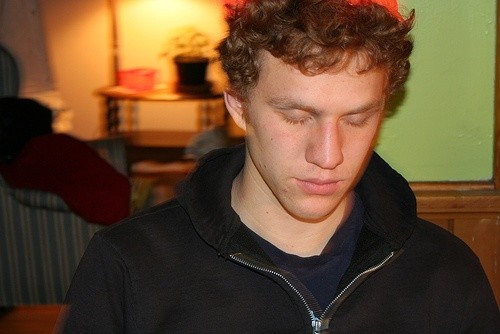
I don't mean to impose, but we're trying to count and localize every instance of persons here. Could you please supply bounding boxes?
[0,97,131,226]
[64,0,500,334]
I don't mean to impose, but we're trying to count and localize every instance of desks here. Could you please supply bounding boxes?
[93,79,231,148]
